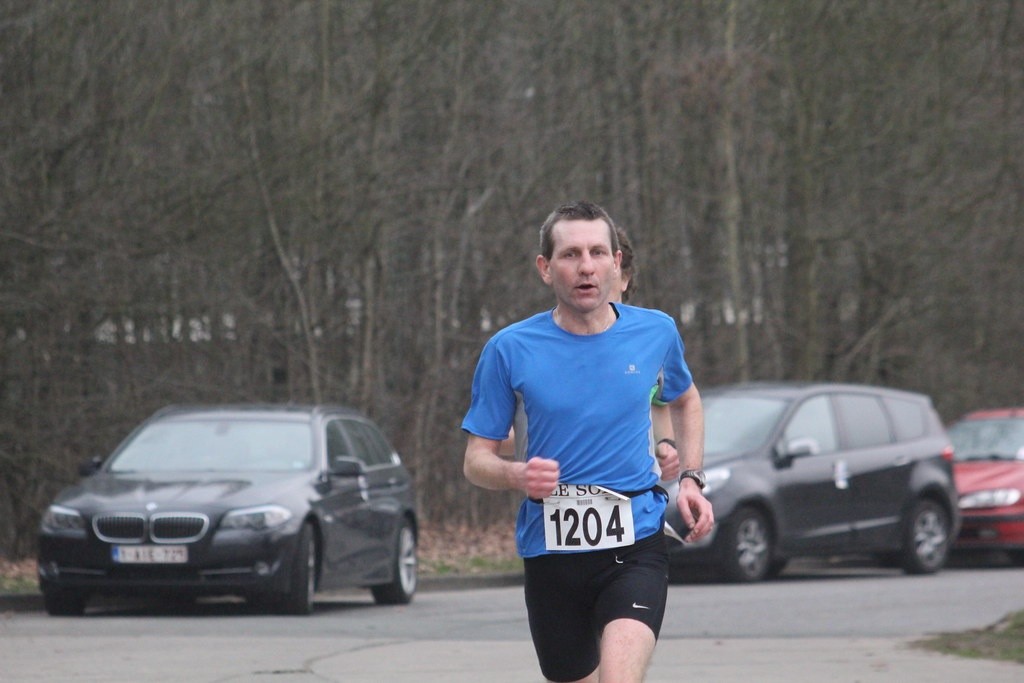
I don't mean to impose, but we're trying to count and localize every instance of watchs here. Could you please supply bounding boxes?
[678,469,707,489]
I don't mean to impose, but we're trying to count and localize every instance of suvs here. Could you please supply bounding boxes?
[645,378,961,586]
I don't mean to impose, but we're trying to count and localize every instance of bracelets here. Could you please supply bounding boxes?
[657,438,676,449]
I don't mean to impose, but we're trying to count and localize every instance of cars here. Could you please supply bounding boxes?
[945,406,1024,572]
[32,397,418,616]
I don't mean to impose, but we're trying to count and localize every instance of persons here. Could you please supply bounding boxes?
[462,201,715,683]
